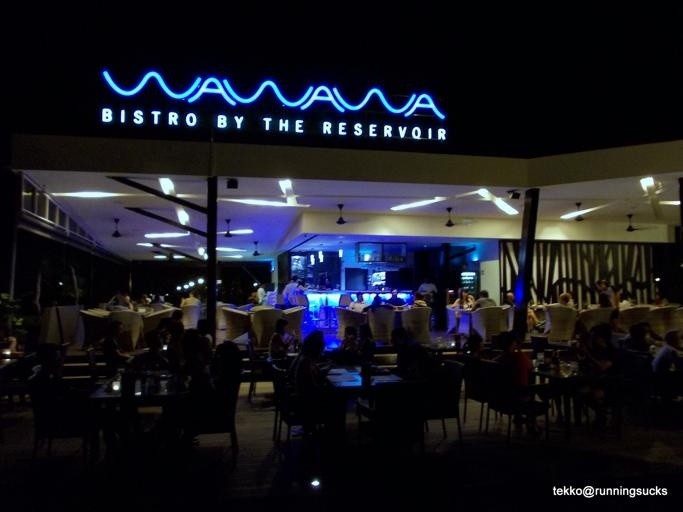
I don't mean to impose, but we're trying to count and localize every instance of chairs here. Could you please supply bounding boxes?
[81,302,178,348]
[262,357,299,439]
[650,303,679,340]
[618,303,650,330]
[165,339,245,450]
[338,294,355,307]
[424,359,465,444]
[181,304,201,332]
[367,309,395,344]
[545,303,578,346]
[471,305,503,340]
[336,306,367,340]
[580,306,616,331]
[283,306,308,342]
[401,307,432,342]
[250,306,282,346]
[219,306,249,340]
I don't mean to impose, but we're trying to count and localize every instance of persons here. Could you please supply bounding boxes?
[388,287,406,305]
[181,291,200,307]
[158,310,184,345]
[452,289,474,308]
[366,297,396,310]
[0,333,17,353]
[104,320,132,377]
[492,331,549,427]
[339,327,357,351]
[145,324,166,350]
[464,335,484,358]
[355,324,376,367]
[104,290,131,305]
[411,293,428,308]
[350,292,367,310]
[418,276,438,314]
[613,285,625,332]
[652,293,669,306]
[583,334,637,407]
[354,329,451,458]
[471,291,496,311]
[283,276,298,308]
[140,294,153,305]
[143,341,242,462]
[174,331,221,385]
[289,331,334,386]
[637,322,663,345]
[27,343,117,465]
[624,326,650,352]
[196,319,212,348]
[291,279,308,306]
[558,293,572,306]
[597,280,615,307]
[588,325,636,364]
[506,292,547,327]
[320,278,332,290]
[151,296,163,310]
[258,281,271,304]
[652,330,683,376]
[270,318,296,360]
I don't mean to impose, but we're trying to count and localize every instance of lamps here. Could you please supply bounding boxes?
[625,214,638,234]
[574,199,584,222]
[318,244,324,263]
[338,241,343,258]
[253,240,262,257]
[310,246,315,266]
[335,203,347,225]
[224,220,234,238]
[110,215,123,239]
[446,206,455,228]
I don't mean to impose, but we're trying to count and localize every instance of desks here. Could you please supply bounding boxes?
[90,371,179,414]
[530,360,581,417]
[344,303,407,315]
[320,367,402,427]
